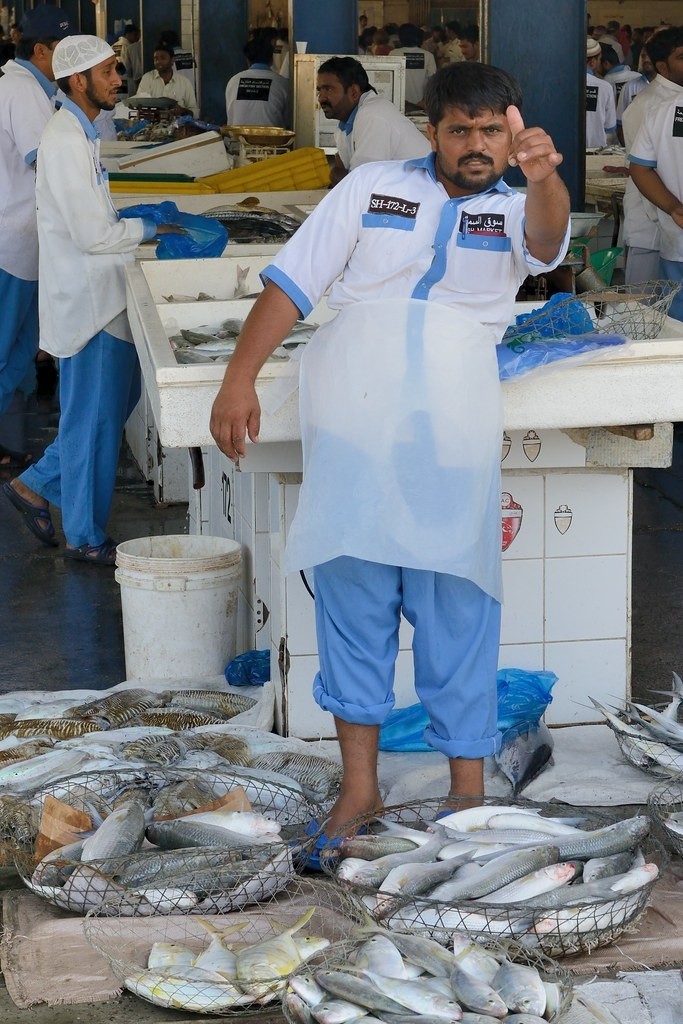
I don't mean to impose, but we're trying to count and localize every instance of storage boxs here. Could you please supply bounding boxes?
[101,130,683,447]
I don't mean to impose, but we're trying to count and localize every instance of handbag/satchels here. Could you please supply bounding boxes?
[119,201,229,260]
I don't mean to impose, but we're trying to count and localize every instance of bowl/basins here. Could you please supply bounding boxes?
[568,236,624,288]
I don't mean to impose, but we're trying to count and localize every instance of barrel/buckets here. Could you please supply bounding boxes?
[114,534,243,683]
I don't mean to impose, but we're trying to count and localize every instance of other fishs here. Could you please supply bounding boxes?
[0,671,683,1024]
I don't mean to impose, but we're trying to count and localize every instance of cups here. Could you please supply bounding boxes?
[296,41,308,55]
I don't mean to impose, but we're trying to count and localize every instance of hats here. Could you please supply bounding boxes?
[21,4,74,36]
[586,38,601,57]
[420,25,432,32]
[52,35,116,80]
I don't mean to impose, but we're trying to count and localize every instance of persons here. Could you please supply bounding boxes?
[317,57,432,186]
[586,21,655,148]
[0,5,184,564]
[209,64,571,869]
[136,45,201,119]
[225,27,290,147]
[358,15,480,113]
[622,29,683,322]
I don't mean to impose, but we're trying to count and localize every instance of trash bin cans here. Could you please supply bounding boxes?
[115,535,243,679]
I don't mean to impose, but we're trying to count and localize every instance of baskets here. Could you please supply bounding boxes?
[606,702,683,771]
[505,279,681,356]
[651,783,683,848]
[81,872,367,1013]
[6,766,327,915]
[280,930,575,1024]
[321,794,670,954]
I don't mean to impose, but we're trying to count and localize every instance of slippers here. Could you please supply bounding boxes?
[299,815,370,871]
[3,481,58,547]
[0,453,33,468]
[62,538,122,565]
